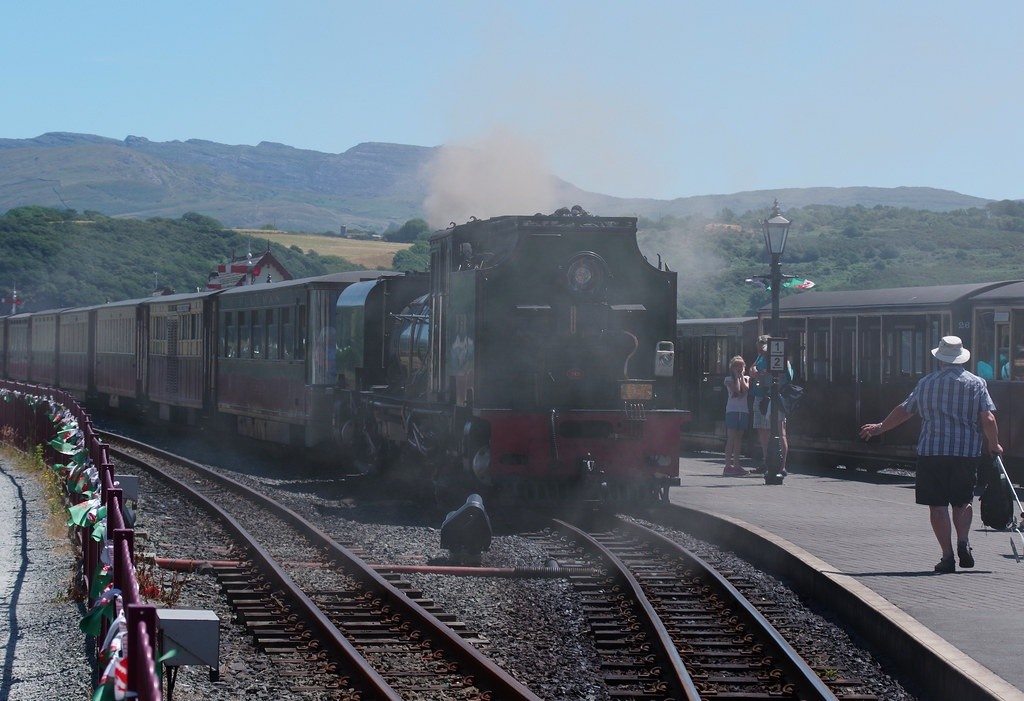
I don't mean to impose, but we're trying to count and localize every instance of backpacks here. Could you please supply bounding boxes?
[978,452,1017,532]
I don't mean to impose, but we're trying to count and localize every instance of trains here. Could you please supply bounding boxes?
[0,201,691,505]
[675,278,1024,480]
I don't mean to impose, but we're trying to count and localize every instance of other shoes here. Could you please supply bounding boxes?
[750,464,766,474]
[724,464,749,476]
[782,470,787,476]
[957,543,974,568]
[935,558,955,572]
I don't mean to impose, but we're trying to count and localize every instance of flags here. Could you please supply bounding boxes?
[784,278,816,290]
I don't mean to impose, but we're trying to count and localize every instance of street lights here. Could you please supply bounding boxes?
[759,195,795,484]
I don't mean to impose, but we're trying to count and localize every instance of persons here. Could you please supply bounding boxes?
[723,356,751,476]
[748,335,793,476]
[979,343,1009,381]
[860,335,1002,573]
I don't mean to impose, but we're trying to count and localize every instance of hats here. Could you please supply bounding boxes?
[756,335,770,342]
[930,335,971,365]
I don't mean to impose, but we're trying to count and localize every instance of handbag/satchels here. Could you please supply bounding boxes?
[778,381,804,418]
